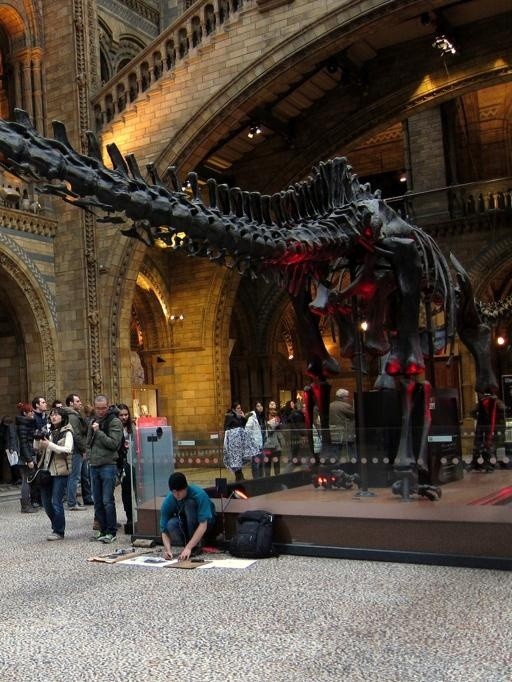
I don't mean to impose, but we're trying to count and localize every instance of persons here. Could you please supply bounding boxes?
[469,389,506,465]
[1,393,138,544]
[328,388,357,464]
[221,399,323,483]
[157,472,222,563]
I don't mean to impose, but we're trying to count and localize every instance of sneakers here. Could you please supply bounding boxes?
[191,547,202,556]
[21,504,122,543]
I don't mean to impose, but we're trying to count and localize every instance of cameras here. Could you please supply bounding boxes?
[34,429,51,440]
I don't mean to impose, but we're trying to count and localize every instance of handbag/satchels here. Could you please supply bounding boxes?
[17,455,37,466]
[229,510,274,560]
[27,470,52,489]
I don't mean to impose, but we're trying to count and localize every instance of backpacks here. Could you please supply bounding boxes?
[93,414,127,468]
